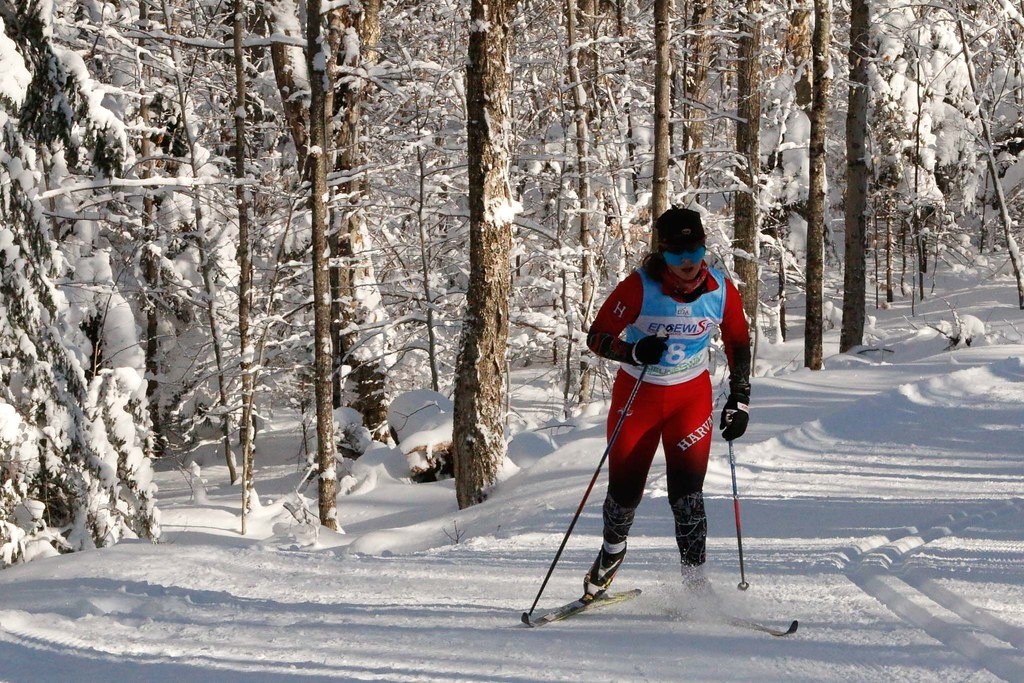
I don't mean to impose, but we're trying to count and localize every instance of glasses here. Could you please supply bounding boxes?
[661,236,706,254]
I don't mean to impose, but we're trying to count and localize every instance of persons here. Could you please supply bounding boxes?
[582,208,752,599]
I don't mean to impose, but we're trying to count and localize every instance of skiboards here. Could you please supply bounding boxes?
[520,588,799,637]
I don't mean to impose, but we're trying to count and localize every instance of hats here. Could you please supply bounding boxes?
[655,204,704,243]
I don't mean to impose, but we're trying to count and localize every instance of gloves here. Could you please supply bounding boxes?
[635,334,670,364]
[720,393,750,442]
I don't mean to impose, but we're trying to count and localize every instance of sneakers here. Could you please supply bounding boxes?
[584,541,627,597]
[682,567,718,601]
